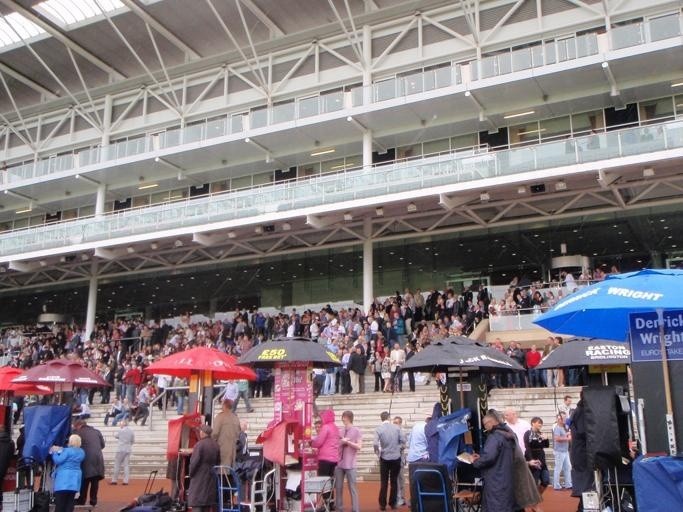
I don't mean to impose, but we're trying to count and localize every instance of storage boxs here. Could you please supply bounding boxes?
[3,502,29,512]
[581,490,600,511]
[3,488,29,502]
[305,476,331,492]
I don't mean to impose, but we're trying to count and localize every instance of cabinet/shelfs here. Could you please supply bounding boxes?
[283,452,317,512]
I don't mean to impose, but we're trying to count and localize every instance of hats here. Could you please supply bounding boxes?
[198,424,214,435]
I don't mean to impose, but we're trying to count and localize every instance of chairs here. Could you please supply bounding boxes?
[240,469,275,512]
[305,474,336,512]
[453,478,484,512]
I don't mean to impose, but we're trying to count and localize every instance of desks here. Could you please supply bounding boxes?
[409,458,455,512]
[178,446,264,501]
[631,452,682,512]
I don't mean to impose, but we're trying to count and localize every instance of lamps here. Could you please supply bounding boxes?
[126,239,184,253]
[344,202,416,221]
[480,181,567,201]
[643,165,655,176]
[228,223,291,238]
[40,254,89,267]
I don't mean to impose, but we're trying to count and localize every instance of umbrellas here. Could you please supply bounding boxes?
[533,268,683,461]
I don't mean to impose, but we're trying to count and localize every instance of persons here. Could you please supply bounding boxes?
[310,409,341,512]
[188,425,221,512]
[314,421,322,434]
[109,421,134,485]
[16,426,30,489]
[392,417,408,507]
[0,265,620,426]
[239,419,249,456]
[335,410,362,512]
[49,435,85,512]
[469,396,576,512]
[406,417,430,507]
[0,424,15,512]
[211,399,241,504]
[374,411,406,511]
[74,420,105,505]
